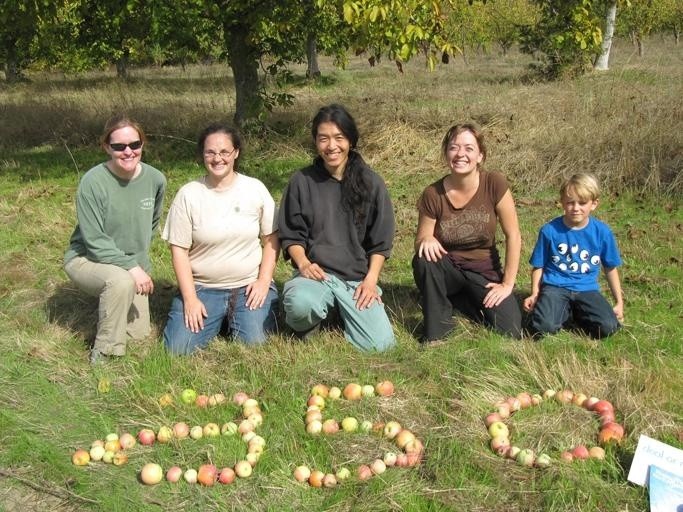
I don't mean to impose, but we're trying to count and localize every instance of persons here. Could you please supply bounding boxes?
[160,121,279,357]
[412,123,521,348]
[521,173,624,342]
[277,103,396,356]
[63,113,166,371]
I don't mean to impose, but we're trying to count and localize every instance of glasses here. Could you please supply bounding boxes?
[204,148,235,158]
[109,140,142,150]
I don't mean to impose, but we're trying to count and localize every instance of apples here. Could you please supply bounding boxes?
[485,388,625,467]
[72,388,265,486]
[293,380,423,487]
